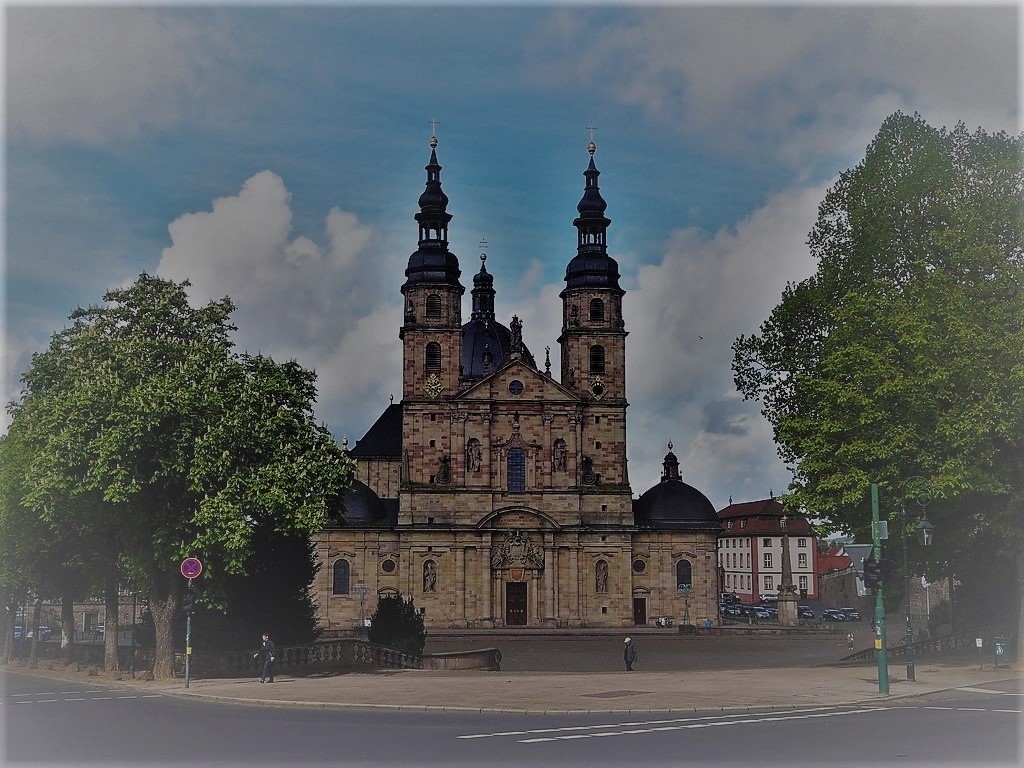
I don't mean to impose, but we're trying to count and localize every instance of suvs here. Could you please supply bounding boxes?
[759,593,778,604]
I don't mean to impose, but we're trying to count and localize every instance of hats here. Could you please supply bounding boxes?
[623,637,631,643]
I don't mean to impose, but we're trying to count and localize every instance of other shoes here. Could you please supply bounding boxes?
[849,648,850,650]
[259,680,264,683]
[266,680,273,683]
[851,648,853,650]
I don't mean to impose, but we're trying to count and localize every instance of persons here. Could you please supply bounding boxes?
[623,637,637,671]
[254,632,276,684]
[847,632,856,651]
[706,619,712,632]
[871,618,876,632]
[657,618,670,629]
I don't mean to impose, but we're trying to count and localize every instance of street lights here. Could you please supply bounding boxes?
[901,476,935,682]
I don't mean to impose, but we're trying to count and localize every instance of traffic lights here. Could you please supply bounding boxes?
[863,559,899,588]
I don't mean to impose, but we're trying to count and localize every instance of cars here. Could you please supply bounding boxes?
[14,627,22,640]
[27,626,51,641]
[96,626,104,635]
[797,605,815,619]
[719,592,778,619]
[823,608,860,623]
